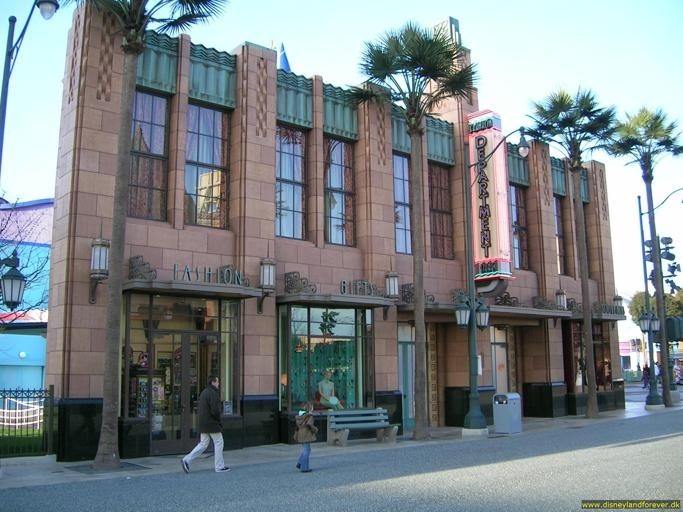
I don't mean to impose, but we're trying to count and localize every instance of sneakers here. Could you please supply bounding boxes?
[296,463,301,469]
[181,458,189,472]
[216,467,231,473]
[301,469,312,472]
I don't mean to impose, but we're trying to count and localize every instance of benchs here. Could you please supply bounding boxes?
[326,408,402,447]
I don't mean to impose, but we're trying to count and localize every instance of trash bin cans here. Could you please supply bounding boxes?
[492,392,522,434]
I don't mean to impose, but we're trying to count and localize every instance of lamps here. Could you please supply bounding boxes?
[612,296,623,307]
[384,270,400,319]
[89,239,111,305]
[256,259,277,314]
[556,289,566,309]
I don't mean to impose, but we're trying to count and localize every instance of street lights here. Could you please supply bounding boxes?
[454,126,530,429]
[638,188,683,405]
[0,0,60,177]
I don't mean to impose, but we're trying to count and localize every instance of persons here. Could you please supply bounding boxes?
[293,400,318,473]
[637,362,641,371]
[644,364,650,385]
[180,374,230,474]
[653,361,662,384]
[316,370,344,410]
[641,371,650,389]
